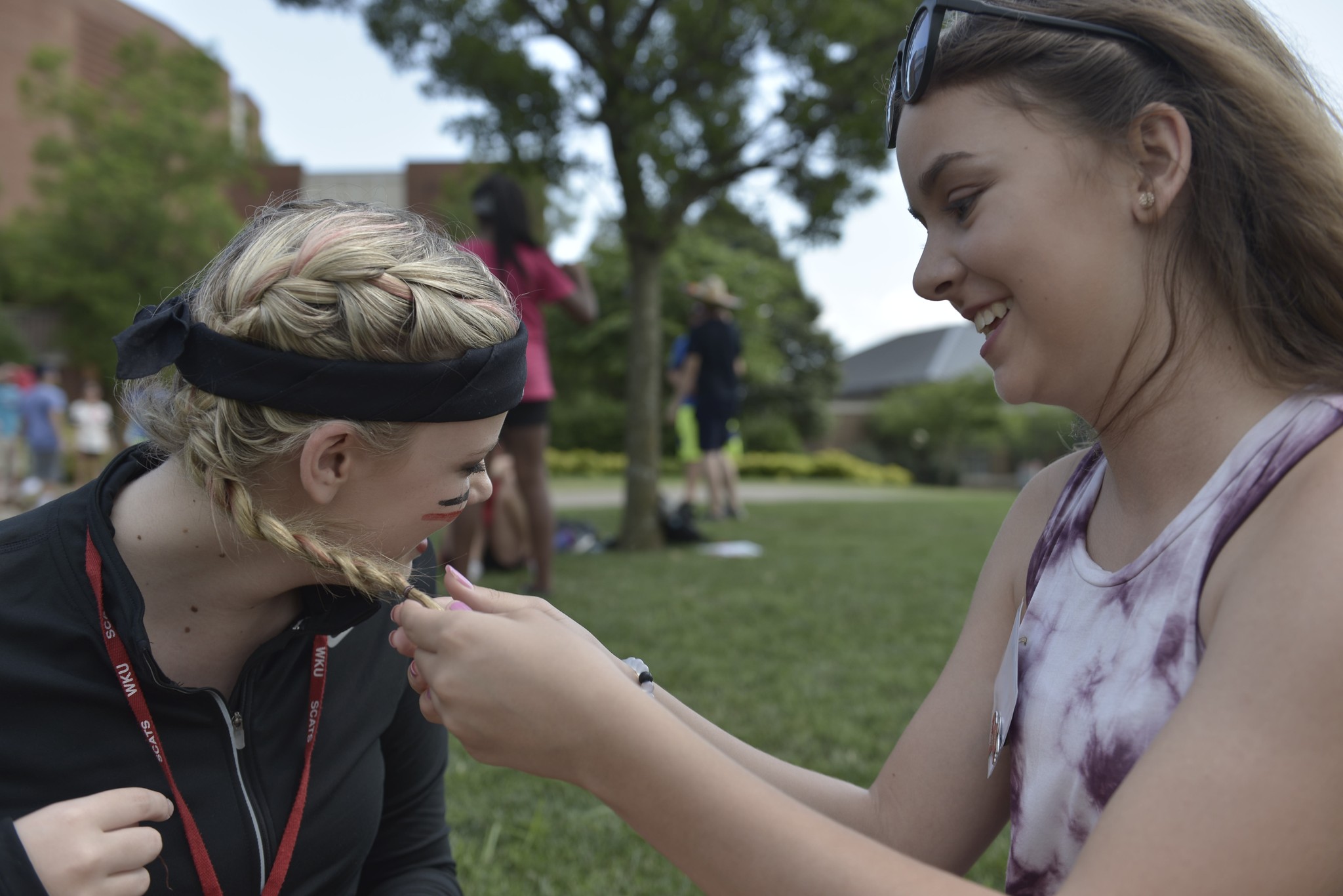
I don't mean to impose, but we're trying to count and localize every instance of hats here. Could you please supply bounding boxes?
[687,274,740,310]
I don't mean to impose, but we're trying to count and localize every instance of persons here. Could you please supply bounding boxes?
[0,359,153,510]
[665,274,748,525]
[1,196,529,896]
[386,1,1343,896]
[439,175,598,604]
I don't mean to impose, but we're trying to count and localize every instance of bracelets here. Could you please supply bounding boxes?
[621,655,654,698]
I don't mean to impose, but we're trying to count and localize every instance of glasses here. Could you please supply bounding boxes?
[886,0,1188,149]
